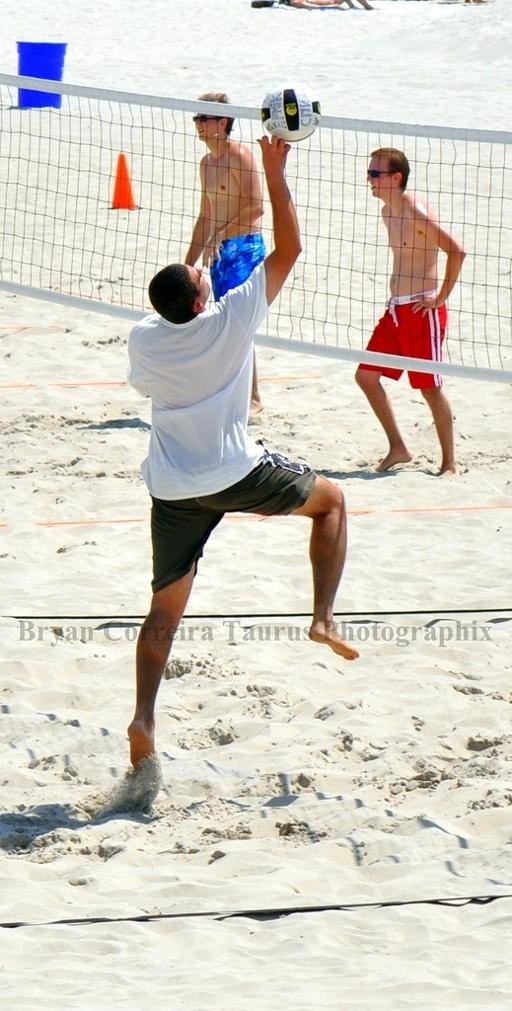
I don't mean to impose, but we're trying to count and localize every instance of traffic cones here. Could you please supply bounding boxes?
[101,150,146,212]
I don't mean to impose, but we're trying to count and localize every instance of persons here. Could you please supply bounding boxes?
[182,93,266,415]
[355,147,468,473]
[127,136,358,764]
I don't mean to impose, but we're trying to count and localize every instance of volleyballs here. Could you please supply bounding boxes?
[259,80,323,142]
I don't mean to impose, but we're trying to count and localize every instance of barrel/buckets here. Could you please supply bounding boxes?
[13,39,68,110]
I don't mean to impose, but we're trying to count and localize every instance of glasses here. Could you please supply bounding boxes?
[192,115,220,122]
[365,168,396,177]
